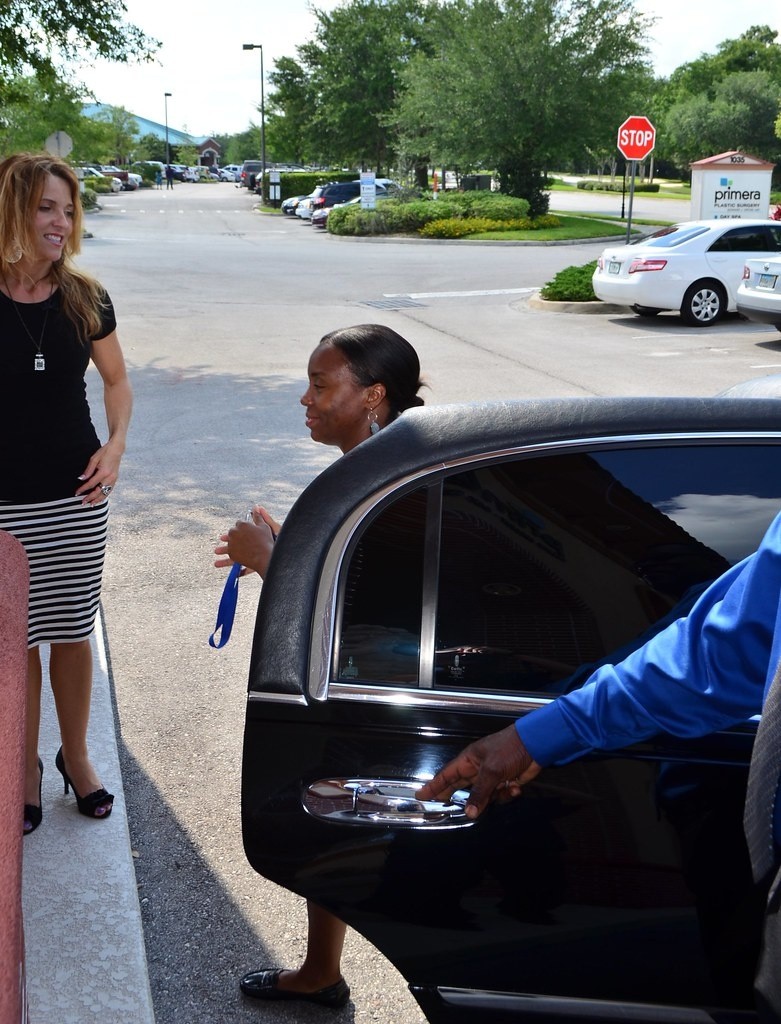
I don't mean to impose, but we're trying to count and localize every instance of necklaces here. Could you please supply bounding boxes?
[0,260,55,370]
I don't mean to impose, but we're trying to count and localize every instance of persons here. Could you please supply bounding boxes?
[414,509,781,1024]
[155,169,162,190]
[165,166,174,190]
[214,323,430,1011]
[0,151,135,838]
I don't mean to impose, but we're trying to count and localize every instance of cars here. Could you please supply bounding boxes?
[591,218,780,327]
[280,178,405,228]
[735,254,781,335]
[75,160,343,192]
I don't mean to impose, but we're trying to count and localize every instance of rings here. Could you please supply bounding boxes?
[98,484,112,496]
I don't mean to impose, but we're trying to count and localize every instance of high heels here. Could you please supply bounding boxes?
[24,755,44,835]
[54,744,114,819]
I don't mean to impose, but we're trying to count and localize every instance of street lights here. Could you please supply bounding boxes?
[242,43,267,179]
[164,92,173,166]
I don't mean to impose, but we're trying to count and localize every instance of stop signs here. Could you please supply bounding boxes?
[617,116,655,162]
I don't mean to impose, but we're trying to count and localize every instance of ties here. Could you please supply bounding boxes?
[742,657,780,882]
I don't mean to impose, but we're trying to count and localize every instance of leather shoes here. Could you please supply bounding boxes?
[240,967,351,1007]
